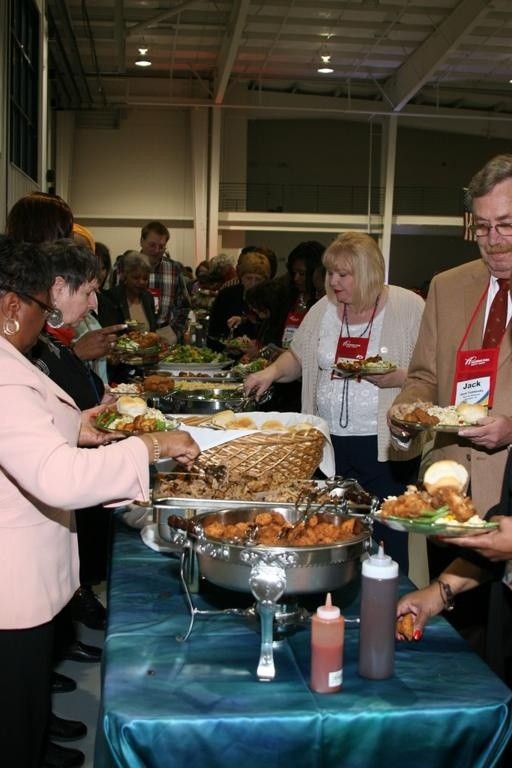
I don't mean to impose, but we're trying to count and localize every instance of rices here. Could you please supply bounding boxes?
[358,360,392,368]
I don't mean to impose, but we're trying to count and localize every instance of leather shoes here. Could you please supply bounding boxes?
[42,591,106,768]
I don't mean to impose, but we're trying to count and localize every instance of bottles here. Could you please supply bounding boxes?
[311,593,344,692]
[184,319,204,348]
[359,541,399,679]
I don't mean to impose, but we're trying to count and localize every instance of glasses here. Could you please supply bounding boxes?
[470,223,512,237]
[0,284,64,330]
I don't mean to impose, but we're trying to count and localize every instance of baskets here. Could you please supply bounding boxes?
[173,417,326,479]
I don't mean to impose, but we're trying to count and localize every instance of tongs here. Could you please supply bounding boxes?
[194,460,227,483]
[225,387,269,412]
[278,474,358,540]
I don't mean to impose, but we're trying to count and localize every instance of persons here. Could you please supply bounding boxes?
[195,155,512,693]
[1,191,196,763]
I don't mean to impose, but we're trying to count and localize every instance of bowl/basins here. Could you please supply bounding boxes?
[127,323,146,332]
[170,392,257,413]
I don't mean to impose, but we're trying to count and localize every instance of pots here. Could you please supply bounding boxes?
[169,504,374,594]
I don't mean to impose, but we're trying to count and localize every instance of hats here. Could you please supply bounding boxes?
[238,253,271,279]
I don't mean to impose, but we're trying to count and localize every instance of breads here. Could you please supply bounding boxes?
[206,407,312,431]
[457,404,487,423]
[425,457,469,497]
[118,396,146,416]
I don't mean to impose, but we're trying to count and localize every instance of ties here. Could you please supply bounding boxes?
[482,278,511,348]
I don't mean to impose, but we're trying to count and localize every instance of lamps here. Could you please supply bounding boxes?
[317,45,334,74]
[134,38,152,67]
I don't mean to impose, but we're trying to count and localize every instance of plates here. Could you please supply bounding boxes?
[391,415,482,433]
[108,392,145,397]
[154,359,236,370]
[330,367,395,374]
[373,511,497,535]
[94,417,181,436]
